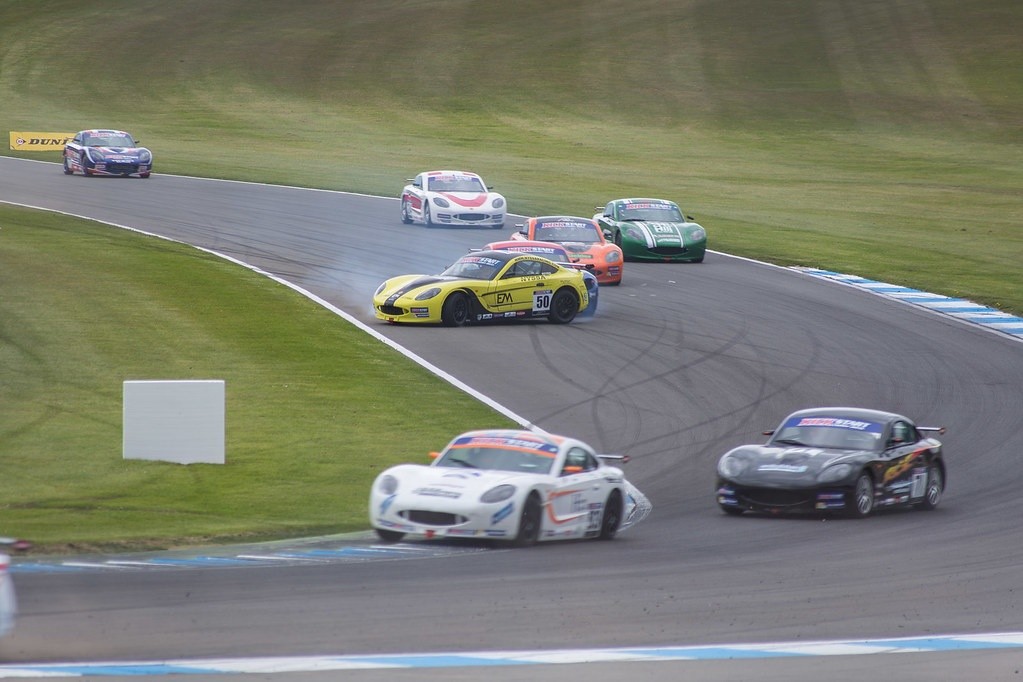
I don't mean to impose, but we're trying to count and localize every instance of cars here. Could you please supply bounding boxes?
[62,129,153,180]
[400,170,508,230]
[372,250,589,328]
[592,197,706,262]
[367,428,653,549]
[468,239,598,317]
[715,407,948,520]
[508,214,623,287]
